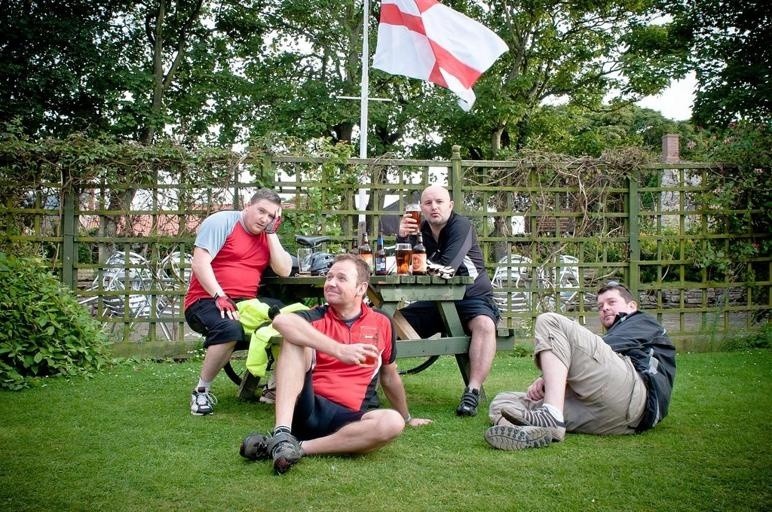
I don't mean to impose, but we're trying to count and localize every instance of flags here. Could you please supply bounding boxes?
[372,0,510,113]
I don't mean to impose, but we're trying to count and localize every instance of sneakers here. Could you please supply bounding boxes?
[238,432,276,461]
[482,423,554,453]
[189,384,215,417]
[259,384,278,405]
[455,387,481,419]
[500,406,566,445]
[266,427,303,473]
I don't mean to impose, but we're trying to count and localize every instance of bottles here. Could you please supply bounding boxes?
[374,231,386,276]
[359,233,374,276]
[349,237,359,257]
[412,233,428,275]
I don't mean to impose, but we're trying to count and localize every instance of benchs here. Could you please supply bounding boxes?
[236,331,284,400]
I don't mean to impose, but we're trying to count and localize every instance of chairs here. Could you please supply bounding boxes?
[491,254,580,313]
[78,252,194,342]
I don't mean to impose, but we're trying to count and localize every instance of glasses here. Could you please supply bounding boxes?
[607,281,632,297]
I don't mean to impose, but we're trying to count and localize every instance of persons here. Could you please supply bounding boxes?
[484,284,676,452]
[239,254,434,476]
[183,187,293,417]
[393,185,500,416]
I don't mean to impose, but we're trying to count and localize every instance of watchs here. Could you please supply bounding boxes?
[214,291,226,301]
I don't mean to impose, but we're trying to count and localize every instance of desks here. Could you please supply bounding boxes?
[236,277,487,407]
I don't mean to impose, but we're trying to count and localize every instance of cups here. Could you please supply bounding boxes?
[406,205,421,236]
[394,243,412,275]
[359,326,378,368]
[297,248,313,276]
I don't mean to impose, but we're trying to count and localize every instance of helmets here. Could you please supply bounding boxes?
[309,251,335,275]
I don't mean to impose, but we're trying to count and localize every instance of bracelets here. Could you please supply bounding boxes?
[405,414,411,424]
